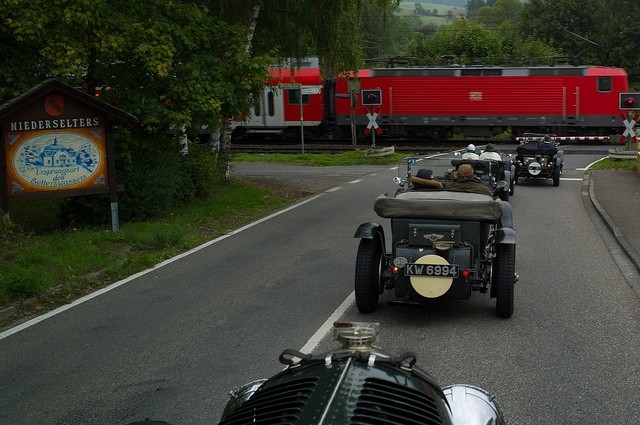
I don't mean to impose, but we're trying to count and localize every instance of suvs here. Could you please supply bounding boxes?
[354,157,520,320]
[216,322,502,422]
[509,133,564,187]
[452,150,510,200]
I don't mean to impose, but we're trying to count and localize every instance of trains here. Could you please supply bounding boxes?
[57,58,630,147]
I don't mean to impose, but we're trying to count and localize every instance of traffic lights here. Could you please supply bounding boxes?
[359,87,386,107]
[618,93,637,111]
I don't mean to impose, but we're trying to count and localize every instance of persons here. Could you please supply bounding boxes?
[544,134,552,143]
[480,143,503,161]
[443,163,492,194]
[461,143,480,162]
[409,168,443,192]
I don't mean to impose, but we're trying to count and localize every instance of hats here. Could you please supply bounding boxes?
[486,142,496,148]
[467,143,476,150]
[456,163,474,175]
